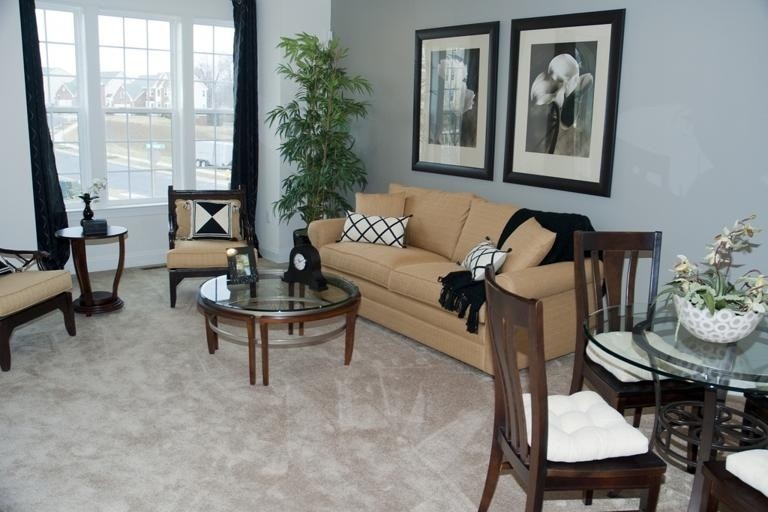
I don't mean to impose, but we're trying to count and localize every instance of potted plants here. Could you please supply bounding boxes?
[264,32,374,247]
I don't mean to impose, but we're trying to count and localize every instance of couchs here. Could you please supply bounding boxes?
[307,184,606,378]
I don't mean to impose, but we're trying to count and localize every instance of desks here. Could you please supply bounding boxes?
[197,271,361,386]
[54,226,128,317]
[587,303,768,512]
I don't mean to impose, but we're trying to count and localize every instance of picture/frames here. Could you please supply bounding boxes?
[503,7,627,198]
[411,20,500,181]
[225,246,259,284]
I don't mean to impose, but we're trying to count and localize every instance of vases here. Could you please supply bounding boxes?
[83,193,93,220]
[673,294,765,343]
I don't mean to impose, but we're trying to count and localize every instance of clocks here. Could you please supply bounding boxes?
[282,235,329,291]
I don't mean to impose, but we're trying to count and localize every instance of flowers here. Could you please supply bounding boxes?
[65,176,108,199]
[649,213,768,316]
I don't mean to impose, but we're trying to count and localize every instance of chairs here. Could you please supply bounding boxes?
[0,248,76,372]
[570,231,701,474]
[478,264,667,512]
[701,449,768,512]
[168,184,257,309]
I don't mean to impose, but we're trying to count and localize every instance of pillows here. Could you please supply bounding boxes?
[341,185,558,282]
[182,198,240,242]
[0,255,14,276]
